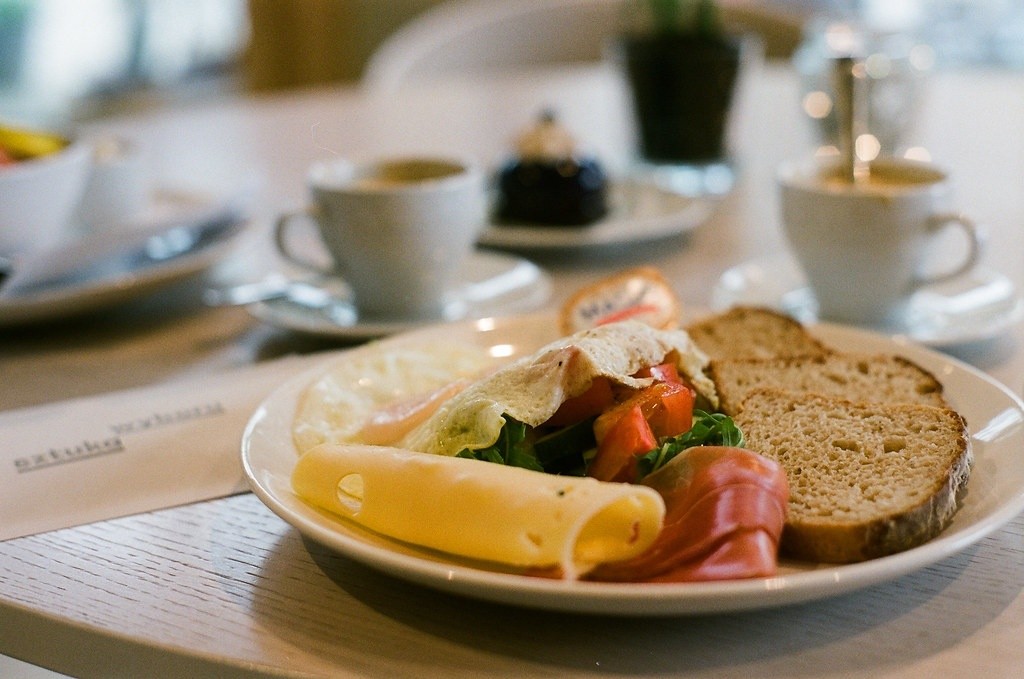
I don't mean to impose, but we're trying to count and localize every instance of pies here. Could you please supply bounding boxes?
[289,319,717,458]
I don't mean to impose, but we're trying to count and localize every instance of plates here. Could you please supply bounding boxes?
[722,250,1018,346]
[2,211,251,323]
[478,163,718,247]
[236,250,556,337]
[239,314,1022,619]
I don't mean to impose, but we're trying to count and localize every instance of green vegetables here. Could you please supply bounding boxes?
[459,409,745,487]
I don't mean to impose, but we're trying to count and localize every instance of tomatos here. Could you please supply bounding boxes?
[536,360,695,478]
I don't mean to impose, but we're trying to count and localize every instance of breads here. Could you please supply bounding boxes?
[691,306,971,566]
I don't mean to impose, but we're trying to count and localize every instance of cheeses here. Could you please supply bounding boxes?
[289,440,667,581]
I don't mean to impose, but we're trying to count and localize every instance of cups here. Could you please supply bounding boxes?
[771,157,981,329]
[276,157,485,314]
